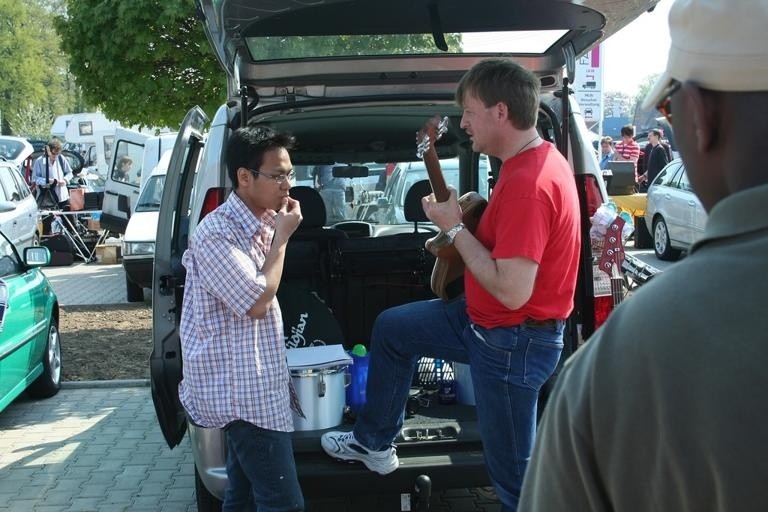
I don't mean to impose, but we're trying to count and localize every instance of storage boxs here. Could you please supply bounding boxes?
[95,244,122,263]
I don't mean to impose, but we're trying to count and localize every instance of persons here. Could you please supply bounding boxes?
[312,164,348,224]
[113,156,132,183]
[615,127,640,193]
[516,0,767,512]
[177,126,304,512]
[32,139,74,242]
[638,130,669,188]
[321,59,581,512]
[599,137,615,172]
[644,128,674,174]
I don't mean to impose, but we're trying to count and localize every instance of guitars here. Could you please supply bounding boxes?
[415,116,488,301]
[598,217,626,308]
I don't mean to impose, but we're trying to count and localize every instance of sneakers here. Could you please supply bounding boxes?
[321,431,399,475]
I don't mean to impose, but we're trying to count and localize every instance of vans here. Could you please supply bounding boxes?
[146,1,634,511]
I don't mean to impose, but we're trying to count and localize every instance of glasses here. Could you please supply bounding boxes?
[51,151,59,155]
[248,169,294,184]
[655,86,679,122]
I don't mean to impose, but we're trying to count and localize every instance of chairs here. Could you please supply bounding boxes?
[289,187,349,242]
[398,179,439,235]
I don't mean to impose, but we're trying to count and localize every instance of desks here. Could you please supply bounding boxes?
[39,209,110,262]
[607,192,647,245]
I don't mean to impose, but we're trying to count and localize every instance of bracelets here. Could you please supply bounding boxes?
[643,174,646,177]
[445,222,467,246]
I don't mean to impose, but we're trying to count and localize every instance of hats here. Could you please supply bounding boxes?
[640,1,768,109]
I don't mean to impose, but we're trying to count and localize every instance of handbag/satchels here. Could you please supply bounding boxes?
[55,185,69,202]
